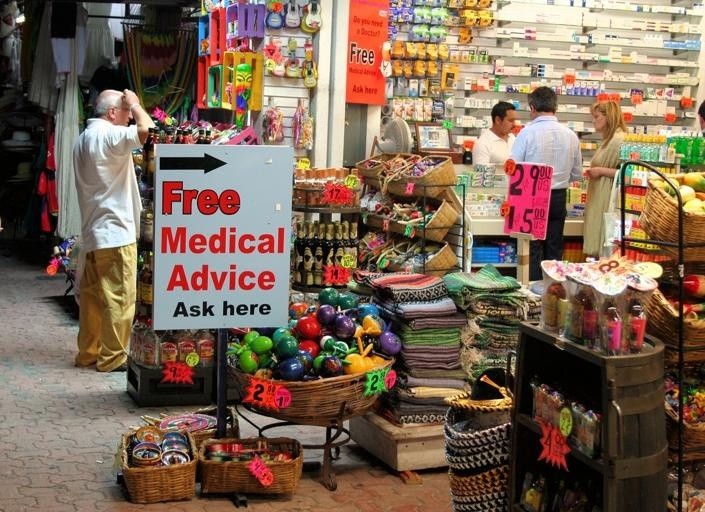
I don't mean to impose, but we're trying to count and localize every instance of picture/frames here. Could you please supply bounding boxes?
[416,122,453,152]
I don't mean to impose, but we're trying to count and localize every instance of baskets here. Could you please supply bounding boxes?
[224,354,396,427]
[625,270,705,364]
[121,404,304,499]
[354,152,463,278]
[440,403,511,508]
[638,175,705,265]
[665,400,705,460]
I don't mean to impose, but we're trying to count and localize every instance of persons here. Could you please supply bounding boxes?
[73,88,156,371]
[510,86,581,280]
[582,100,627,260]
[471,102,517,163]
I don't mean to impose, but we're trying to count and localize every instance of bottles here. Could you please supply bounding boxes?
[620,129,705,313]
[128,125,214,370]
[603,307,622,355]
[627,303,647,352]
[292,168,361,289]
[543,278,646,340]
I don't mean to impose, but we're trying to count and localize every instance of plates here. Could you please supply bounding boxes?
[131,427,190,466]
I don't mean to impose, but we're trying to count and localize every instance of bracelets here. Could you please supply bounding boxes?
[130,102,138,110]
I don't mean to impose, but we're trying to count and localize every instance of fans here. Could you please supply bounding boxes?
[378,117,412,155]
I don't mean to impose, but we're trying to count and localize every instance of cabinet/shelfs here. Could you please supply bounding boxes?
[392,1,705,139]
[508,321,669,512]
[127,174,216,407]
[618,159,705,255]
[292,169,532,294]
